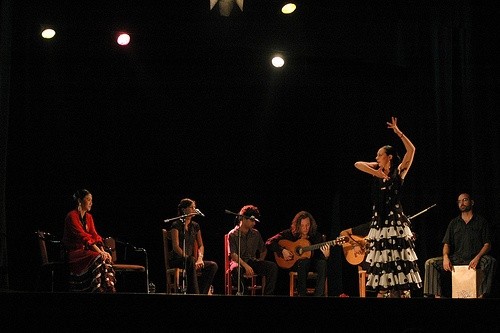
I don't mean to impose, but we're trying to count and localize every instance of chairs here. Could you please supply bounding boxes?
[224,233,266,296]
[357,263,370,298]
[289,234,329,298]
[97,237,151,295]
[36,229,70,294]
[162,228,191,295]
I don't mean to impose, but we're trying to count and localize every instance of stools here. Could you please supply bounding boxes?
[449,265,486,298]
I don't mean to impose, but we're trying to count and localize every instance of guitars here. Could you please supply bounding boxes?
[342,232,416,265]
[274,235,349,269]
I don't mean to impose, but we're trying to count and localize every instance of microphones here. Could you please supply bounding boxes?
[195,209,205,217]
[250,216,260,223]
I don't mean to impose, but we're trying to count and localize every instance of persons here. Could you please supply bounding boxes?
[227,205,278,294]
[64,189,117,293]
[355,117,423,298]
[340,223,371,270]
[167,198,218,295]
[265,211,330,297]
[423,192,496,299]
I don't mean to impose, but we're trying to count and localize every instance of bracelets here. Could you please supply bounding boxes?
[400,132,404,138]
[373,168,376,177]
[199,253,204,257]
